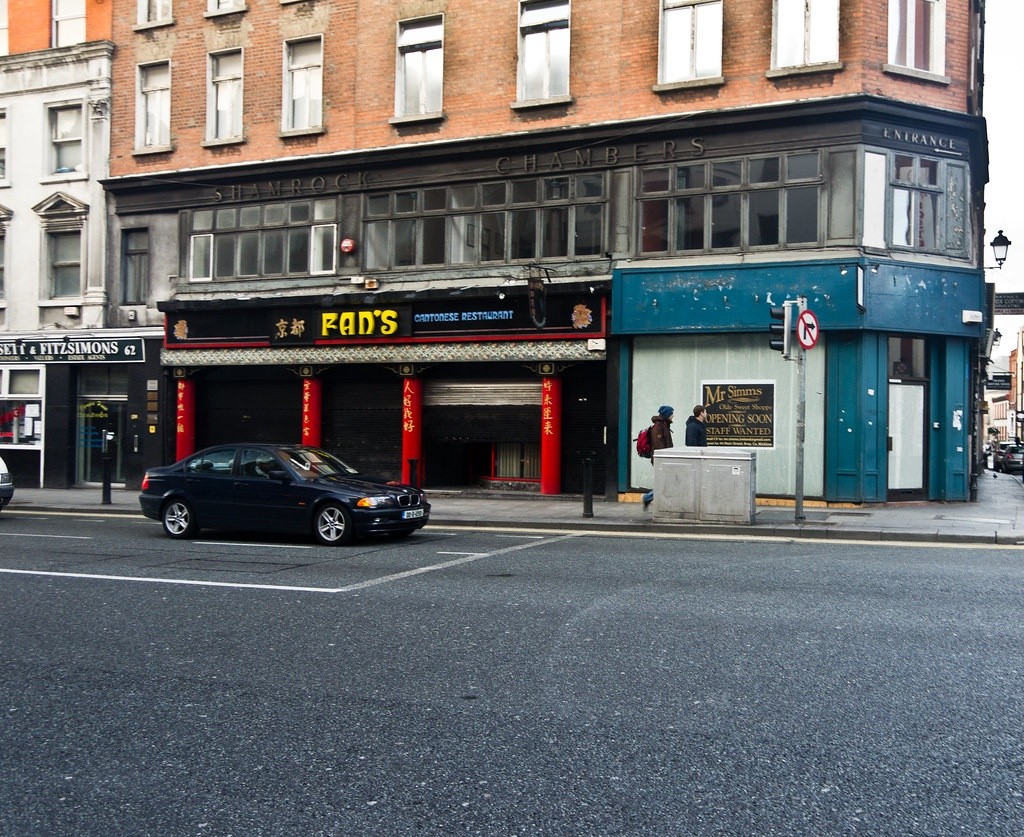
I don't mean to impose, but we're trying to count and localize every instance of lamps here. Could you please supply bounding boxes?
[990,230,1012,269]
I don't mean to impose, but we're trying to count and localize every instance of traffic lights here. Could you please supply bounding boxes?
[770,305,791,359]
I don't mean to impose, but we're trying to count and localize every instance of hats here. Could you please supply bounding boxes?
[658,406,674,416]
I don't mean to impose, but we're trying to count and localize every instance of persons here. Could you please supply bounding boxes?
[685,405,708,447]
[640,405,674,512]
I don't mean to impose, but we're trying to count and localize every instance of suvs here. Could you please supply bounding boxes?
[993,442,1016,473]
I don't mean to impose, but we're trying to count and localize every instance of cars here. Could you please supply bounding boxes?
[1002,445,1023,472]
[0,454,14,511]
[139,442,431,545]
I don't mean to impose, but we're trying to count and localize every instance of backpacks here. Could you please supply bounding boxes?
[636,425,655,458]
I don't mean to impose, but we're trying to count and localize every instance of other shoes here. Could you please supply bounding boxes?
[641,494,647,512]
